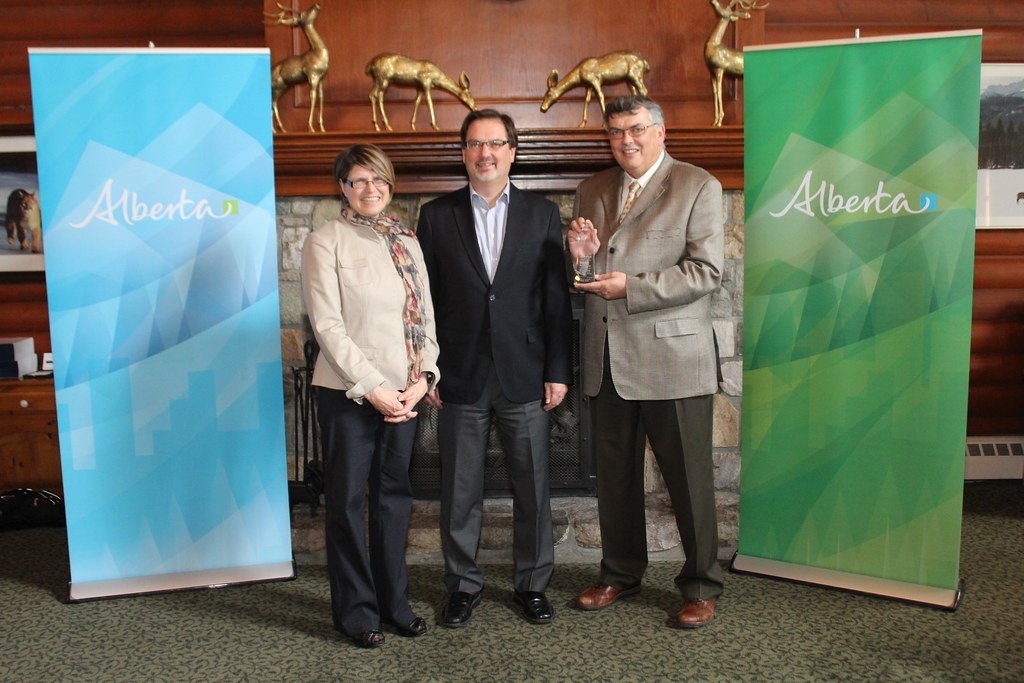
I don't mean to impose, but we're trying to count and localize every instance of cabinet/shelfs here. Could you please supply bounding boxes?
[0,375,63,492]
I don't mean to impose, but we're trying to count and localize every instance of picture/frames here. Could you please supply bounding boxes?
[0,123,48,284]
[976,59,1024,230]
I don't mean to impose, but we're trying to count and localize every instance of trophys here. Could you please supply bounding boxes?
[574,228,596,283]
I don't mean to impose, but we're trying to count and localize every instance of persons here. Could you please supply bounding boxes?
[415,108,573,628]
[565,95,724,627]
[301,142,441,648]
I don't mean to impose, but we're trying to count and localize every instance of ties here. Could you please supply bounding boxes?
[619,182,641,226]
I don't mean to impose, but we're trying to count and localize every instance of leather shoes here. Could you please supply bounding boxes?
[443,592,482,627]
[575,580,642,610]
[513,589,555,623]
[676,598,717,627]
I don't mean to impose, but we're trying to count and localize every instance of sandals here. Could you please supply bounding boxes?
[381,615,427,637]
[351,629,385,648]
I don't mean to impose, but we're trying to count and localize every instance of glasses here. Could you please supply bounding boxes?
[463,139,510,151]
[342,177,390,189]
[607,123,655,139]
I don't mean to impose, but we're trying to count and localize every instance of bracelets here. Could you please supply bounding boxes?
[426,373,433,384]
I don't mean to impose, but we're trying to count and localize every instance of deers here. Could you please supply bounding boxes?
[366,52,478,134]
[262,0,331,134]
[704,0,769,128]
[540,50,652,127]
